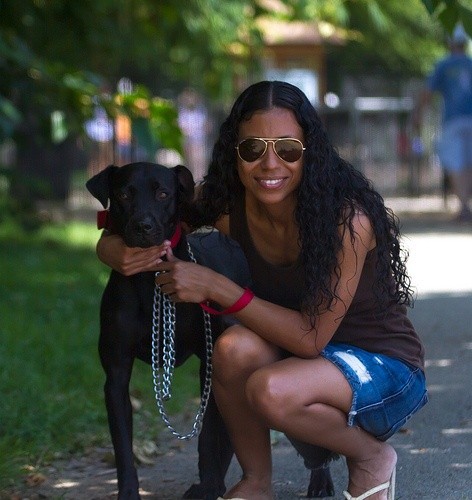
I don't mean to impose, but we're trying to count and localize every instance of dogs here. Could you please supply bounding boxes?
[85,161,336,500]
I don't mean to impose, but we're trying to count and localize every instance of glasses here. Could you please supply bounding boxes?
[234,137,306,164]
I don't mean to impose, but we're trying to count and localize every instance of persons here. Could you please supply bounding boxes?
[410,26,472,220]
[94,80,428,500]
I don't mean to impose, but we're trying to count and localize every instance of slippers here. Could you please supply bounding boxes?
[342,466,396,500]
